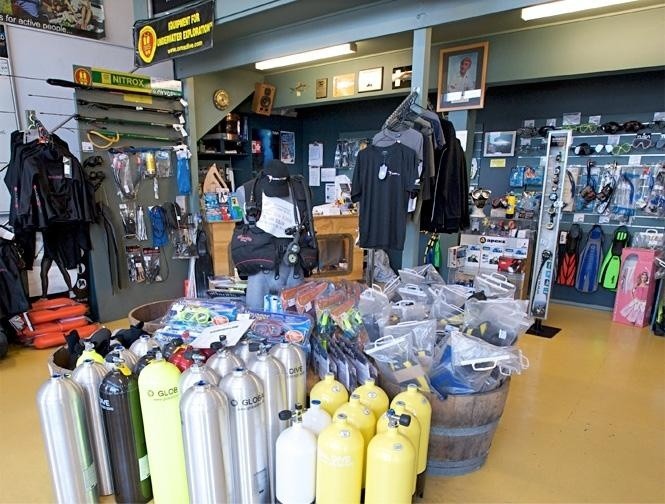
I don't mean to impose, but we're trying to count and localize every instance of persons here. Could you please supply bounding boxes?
[449,55,476,104]
[2,0,104,35]
[235,157,316,310]
[618,265,651,326]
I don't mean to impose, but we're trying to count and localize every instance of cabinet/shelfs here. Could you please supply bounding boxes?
[560,131,665,233]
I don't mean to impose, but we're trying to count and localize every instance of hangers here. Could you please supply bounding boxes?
[370,91,419,147]
[16,113,55,146]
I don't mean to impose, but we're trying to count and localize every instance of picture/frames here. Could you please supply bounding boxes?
[356,66,384,92]
[483,130,517,157]
[333,74,356,96]
[436,41,490,114]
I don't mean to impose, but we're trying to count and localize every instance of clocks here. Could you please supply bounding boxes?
[214,88,232,110]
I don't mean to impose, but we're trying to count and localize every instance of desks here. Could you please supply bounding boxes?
[202,213,364,283]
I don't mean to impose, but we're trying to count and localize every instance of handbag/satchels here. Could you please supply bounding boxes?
[632,229,665,280]
[299,244,319,271]
[231,223,283,275]
[358,264,536,396]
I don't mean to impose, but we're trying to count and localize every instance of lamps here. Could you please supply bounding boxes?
[521,0,636,22]
[255,43,358,71]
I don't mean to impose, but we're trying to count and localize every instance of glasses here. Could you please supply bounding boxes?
[253,323,282,337]
[516,121,665,155]
[89,156,103,167]
[89,171,104,178]
[491,196,508,208]
[180,309,210,324]
[471,187,491,200]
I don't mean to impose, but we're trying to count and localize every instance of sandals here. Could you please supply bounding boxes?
[566,223,581,255]
[611,225,629,257]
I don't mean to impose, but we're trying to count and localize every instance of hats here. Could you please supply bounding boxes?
[261,159,290,197]
